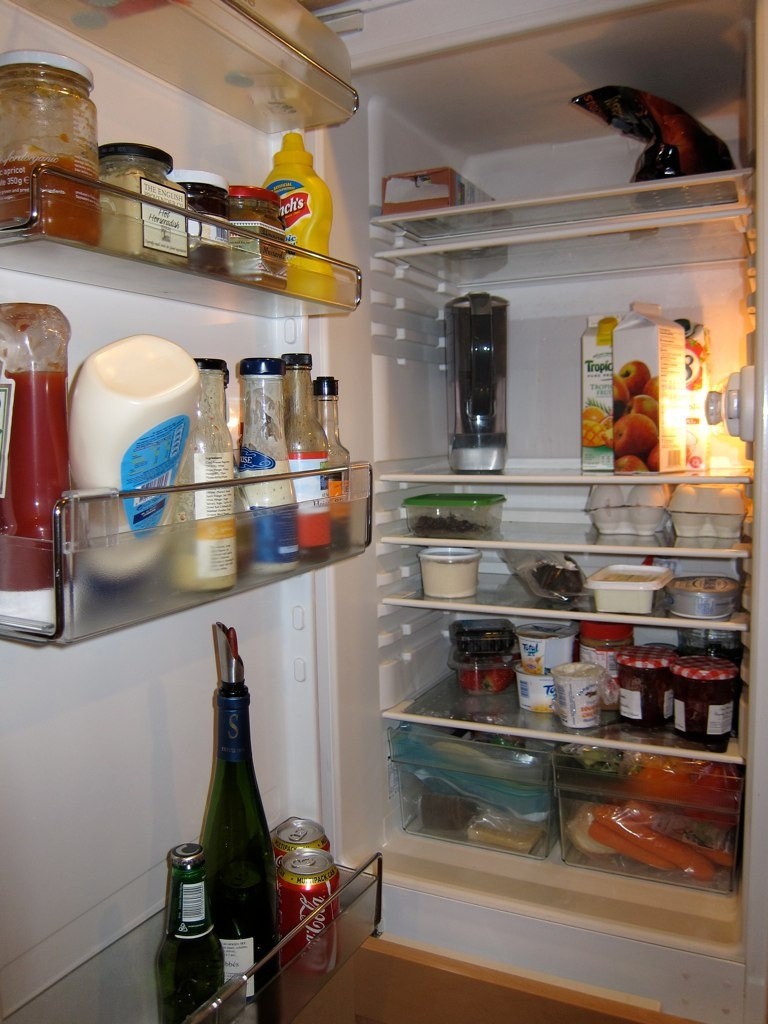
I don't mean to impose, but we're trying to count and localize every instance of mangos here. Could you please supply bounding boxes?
[582,405,614,448]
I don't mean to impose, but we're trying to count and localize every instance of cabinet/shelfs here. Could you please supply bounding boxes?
[382,162,748,954]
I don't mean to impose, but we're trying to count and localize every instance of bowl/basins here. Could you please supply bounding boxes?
[415,547,483,599]
[581,564,674,613]
[515,623,578,673]
[448,617,522,658]
[448,645,519,695]
[514,661,559,711]
[402,492,506,538]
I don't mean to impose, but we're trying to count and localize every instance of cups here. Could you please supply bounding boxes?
[551,662,606,727]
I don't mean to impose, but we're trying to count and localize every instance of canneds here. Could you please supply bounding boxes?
[228,184,290,291]
[669,654,739,739]
[169,169,233,280]
[613,646,679,726]
[274,819,331,863]
[0,48,101,246]
[98,142,190,268]
[276,847,341,977]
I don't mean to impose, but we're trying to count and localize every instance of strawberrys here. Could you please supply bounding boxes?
[483,664,515,694]
[460,670,484,690]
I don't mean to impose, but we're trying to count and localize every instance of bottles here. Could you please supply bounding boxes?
[197,619,282,1024]
[0,303,351,629]
[157,844,223,1022]
[257,131,339,303]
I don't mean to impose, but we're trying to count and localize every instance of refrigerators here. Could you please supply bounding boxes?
[0,0,768,1024]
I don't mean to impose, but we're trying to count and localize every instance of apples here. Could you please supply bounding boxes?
[613,360,659,471]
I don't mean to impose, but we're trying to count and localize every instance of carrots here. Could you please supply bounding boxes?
[590,800,734,880]
